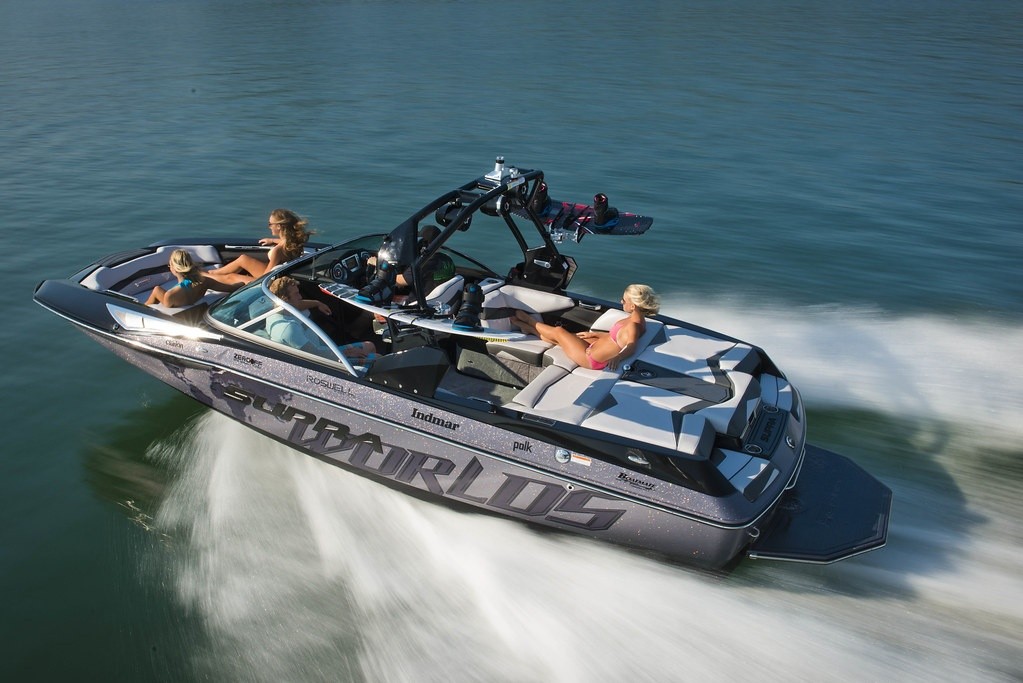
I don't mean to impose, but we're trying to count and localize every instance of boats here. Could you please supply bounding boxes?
[29,156,894,574]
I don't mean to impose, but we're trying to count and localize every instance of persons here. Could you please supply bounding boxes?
[367,225,455,306]
[199,209,311,285]
[145,249,208,309]
[509,284,660,371]
[266,277,383,370]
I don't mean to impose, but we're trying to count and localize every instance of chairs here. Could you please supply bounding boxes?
[409,275,464,311]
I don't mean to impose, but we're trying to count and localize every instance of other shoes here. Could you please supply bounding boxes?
[593,192,620,229]
[356,261,398,304]
[531,182,551,215]
[447,283,484,331]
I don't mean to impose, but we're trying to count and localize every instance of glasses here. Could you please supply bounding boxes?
[268,223,277,226]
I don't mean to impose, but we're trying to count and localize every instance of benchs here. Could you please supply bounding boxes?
[81,245,221,291]
[147,289,230,316]
[513,308,664,425]
